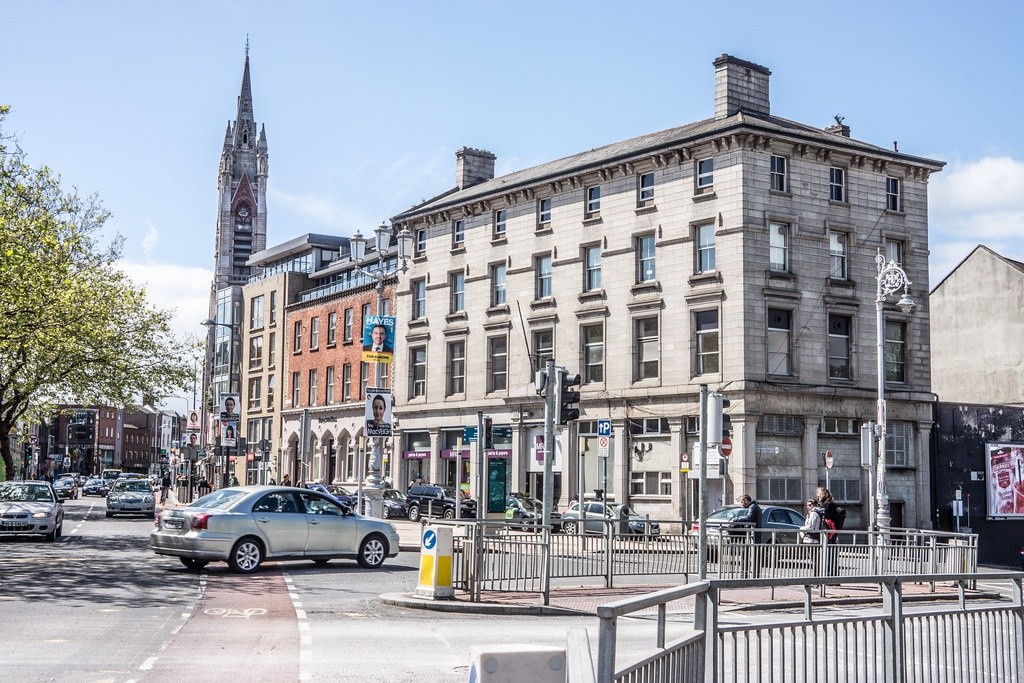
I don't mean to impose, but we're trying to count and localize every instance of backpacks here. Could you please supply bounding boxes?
[828,500,847,530]
[817,511,838,541]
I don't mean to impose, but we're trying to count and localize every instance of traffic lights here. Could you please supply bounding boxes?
[484,416,494,449]
[26,445,32,460]
[555,369,581,425]
[706,393,731,445]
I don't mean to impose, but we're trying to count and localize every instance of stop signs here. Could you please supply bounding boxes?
[721,437,733,456]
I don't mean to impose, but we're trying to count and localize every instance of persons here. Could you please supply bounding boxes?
[89,473,101,479]
[383,478,392,499]
[198,476,215,494]
[189,434,198,446]
[730,495,763,578]
[296,480,308,489]
[363,324,393,353]
[159,472,172,503]
[189,412,199,425]
[225,398,235,415]
[171,458,178,465]
[229,474,239,487]
[268,478,275,485]
[226,425,235,438]
[407,481,414,494]
[176,475,196,498]
[371,394,387,427]
[800,487,840,586]
[280,474,291,486]
[31,474,60,483]
[172,442,179,454]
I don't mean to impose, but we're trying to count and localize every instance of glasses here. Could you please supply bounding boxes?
[808,505,813,506]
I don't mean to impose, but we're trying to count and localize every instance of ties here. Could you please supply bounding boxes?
[376,347,380,351]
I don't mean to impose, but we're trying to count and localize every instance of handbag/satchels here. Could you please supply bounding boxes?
[728,515,746,540]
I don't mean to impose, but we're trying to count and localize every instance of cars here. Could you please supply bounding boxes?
[687,503,806,568]
[149,482,400,575]
[104,477,160,519]
[301,483,410,521]
[561,499,660,541]
[0,479,65,542]
[51,469,201,500]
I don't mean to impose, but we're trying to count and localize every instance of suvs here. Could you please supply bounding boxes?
[505,491,562,534]
[405,484,477,522]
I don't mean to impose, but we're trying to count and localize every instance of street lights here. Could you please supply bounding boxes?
[200,318,234,487]
[160,394,188,419]
[350,220,417,516]
[875,245,916,572]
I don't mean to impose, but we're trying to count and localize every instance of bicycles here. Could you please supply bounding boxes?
[160,486,173,506]
